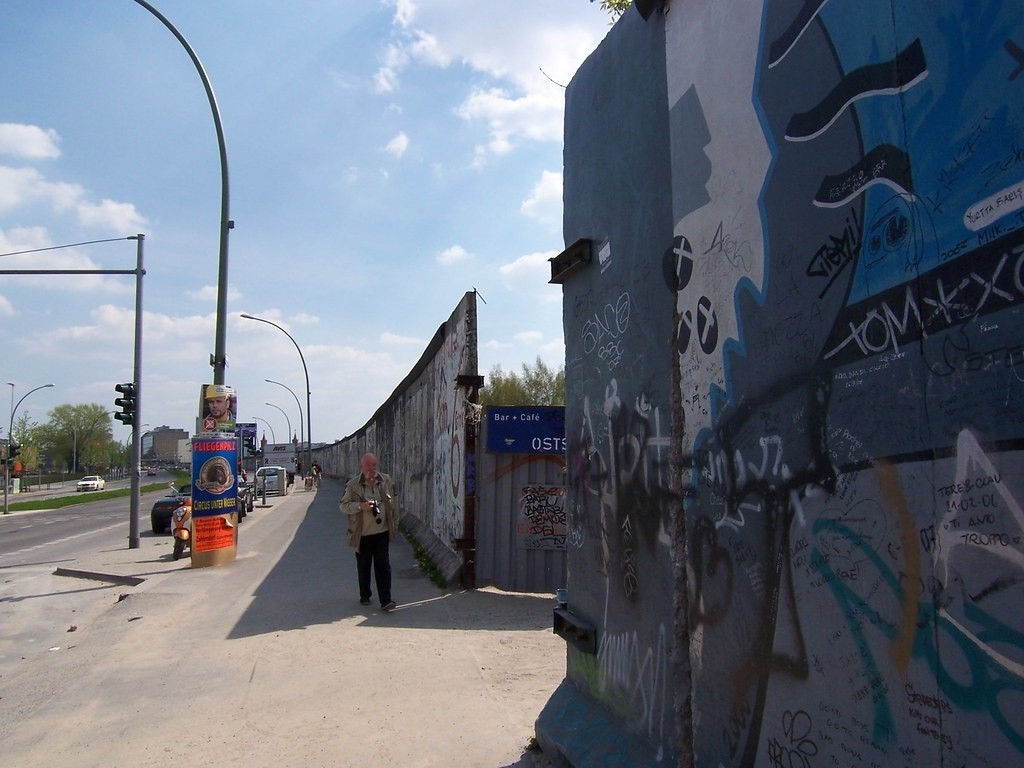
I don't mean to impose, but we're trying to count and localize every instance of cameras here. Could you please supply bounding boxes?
[369,500,381,518]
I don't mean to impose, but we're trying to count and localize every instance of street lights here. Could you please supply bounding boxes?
[251,417,275,444]
[3,383,57,515]
[264,379,304,480]
[87,409,118,476]
[6,382,15,485]
[265,402,292,443]
[126,423,149,474]
[239,313,313,477]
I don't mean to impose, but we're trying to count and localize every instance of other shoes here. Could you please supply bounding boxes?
[381,601,396,610]
[360,596,370,605]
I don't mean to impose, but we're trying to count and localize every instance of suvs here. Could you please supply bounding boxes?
[256,466,282,496]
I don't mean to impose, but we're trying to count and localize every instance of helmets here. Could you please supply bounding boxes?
[204,385,231,399]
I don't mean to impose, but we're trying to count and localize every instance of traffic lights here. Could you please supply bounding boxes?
[8,444,21,459]
[243,435,256,456]
[114,382,137,427]
[7,458,14,465]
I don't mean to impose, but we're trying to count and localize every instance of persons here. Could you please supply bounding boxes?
[297,461,302,475]
[339,453,399,611]
[204,385,235,433]
[312,460,322,486]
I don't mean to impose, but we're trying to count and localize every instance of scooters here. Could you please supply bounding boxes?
[168,480,191,561]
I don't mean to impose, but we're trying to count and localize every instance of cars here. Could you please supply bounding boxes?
[150,483,192,533]
[76,476,106,492]
[236,475,256,523]
[147,468,156,476]
